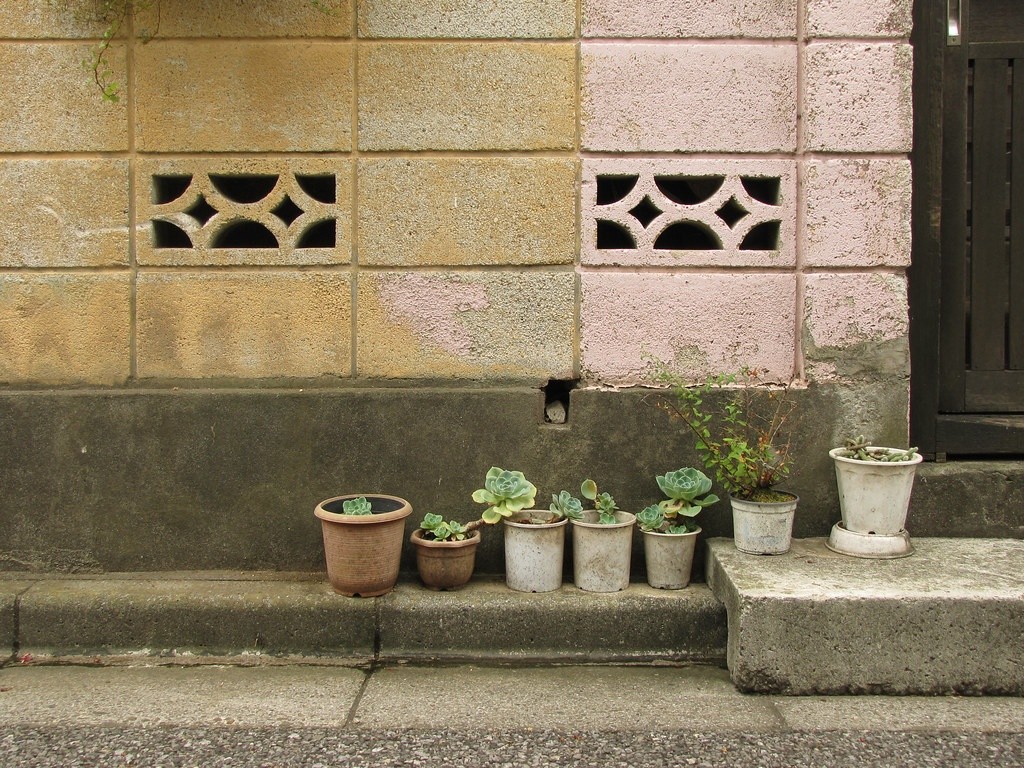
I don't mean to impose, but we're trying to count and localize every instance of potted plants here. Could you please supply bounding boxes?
[314,494,413,598]
[638,343,800,555]
[635,468,720,589]
[410,466,537,591]
[823,434,923,560]
[569,479,637,594]
[503,490,585,593]
